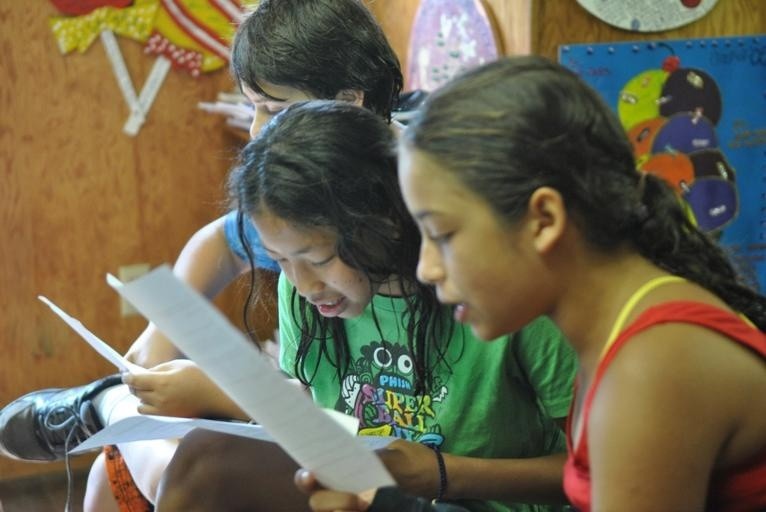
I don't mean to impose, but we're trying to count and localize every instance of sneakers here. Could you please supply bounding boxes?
[0,369,128,511]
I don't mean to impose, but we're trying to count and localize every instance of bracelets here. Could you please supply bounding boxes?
[423,441,450,506]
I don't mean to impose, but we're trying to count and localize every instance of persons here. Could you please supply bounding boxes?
[156,96,583,511]
[0,1,431,512]
[294,55,765,511]
